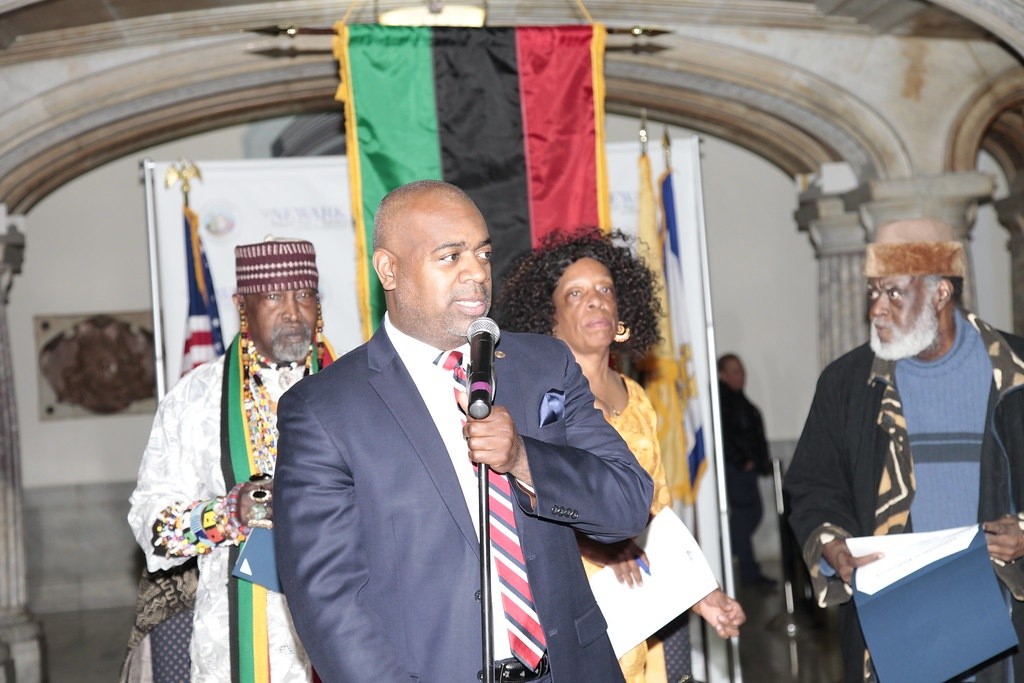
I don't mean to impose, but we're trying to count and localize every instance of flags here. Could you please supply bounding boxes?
[183,210,225,372]
[345,23,607,339]
[640,161,708,514]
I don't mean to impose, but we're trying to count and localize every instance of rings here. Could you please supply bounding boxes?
[248,473,273,530]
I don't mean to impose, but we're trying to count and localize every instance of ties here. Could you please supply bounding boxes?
[434,350,546,671]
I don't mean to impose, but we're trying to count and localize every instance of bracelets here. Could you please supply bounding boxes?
[155,485,250,557]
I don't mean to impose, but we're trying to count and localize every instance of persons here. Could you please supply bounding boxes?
[272,180,654,683]
[497,227,744,683]
[125,240,342,683]
[783,219,1024,683]
[715,353,774,585]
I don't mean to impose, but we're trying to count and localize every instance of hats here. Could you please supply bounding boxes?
[235,239,318,294]
[864,220,966,277]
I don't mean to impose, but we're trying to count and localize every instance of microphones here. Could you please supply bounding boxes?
[466,317,500,420]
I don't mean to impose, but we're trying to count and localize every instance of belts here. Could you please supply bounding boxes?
[495,655,549,683]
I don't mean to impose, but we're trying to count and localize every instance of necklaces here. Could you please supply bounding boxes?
[610,406,620,421]
[247,344,310,482]
